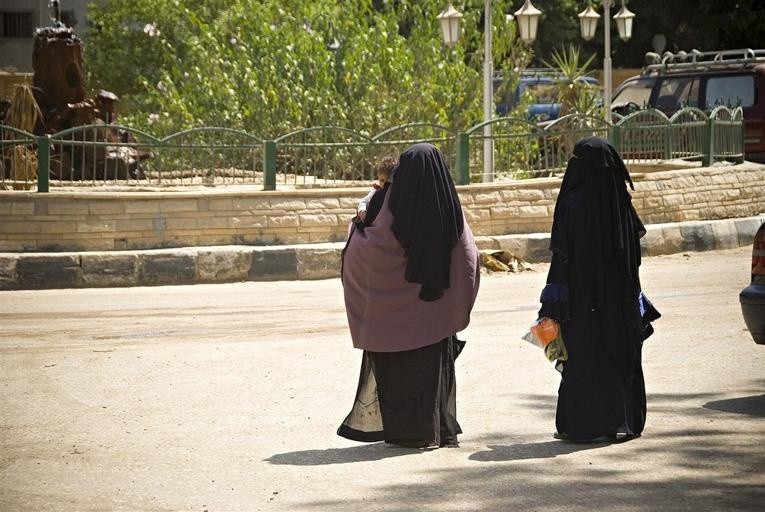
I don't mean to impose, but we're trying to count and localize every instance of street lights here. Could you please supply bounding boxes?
[435,1,543,183]
[577,0,637,142]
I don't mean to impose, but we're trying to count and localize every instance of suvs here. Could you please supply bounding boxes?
[595,47,765,163]
[491,67,604,133]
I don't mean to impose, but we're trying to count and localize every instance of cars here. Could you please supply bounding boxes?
[739,222,765,346]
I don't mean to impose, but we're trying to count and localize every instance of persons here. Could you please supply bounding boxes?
[530,136,645,443]
[338,143,470,450]
[357,155,399,225]
